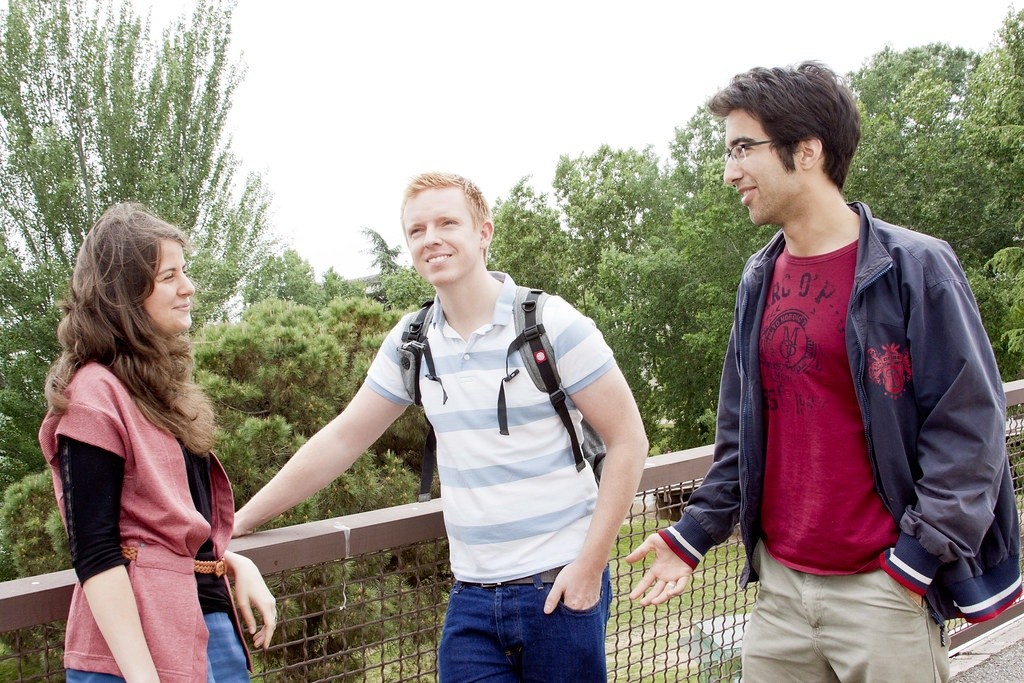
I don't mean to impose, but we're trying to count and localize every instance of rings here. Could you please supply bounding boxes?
[666,582,677,589]
[273,615,279,622]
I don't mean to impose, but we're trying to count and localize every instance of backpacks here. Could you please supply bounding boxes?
[401,287,605,502]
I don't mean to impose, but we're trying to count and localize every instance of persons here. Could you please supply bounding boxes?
[623,59,1023,683]
[228,167,651,683]
[40,200,278,683]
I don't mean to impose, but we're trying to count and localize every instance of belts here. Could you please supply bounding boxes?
[459,565,564,587]
[122,545,227,579]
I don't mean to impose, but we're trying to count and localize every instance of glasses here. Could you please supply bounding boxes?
[726,136,773,162]
